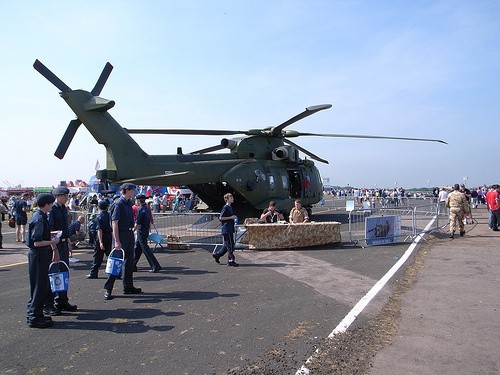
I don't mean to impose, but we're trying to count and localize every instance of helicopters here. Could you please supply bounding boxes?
[33,57,446,230]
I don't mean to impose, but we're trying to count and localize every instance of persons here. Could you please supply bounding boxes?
[477,185,500,231]
[46,187,78,316]
[432,184,478,226]
[25,193,61,327]
[103,183,142,300]
[330,186,405,209]
[66,192,190,250]
[0,192,31,249]
[212,193,239,267]
[260,201,284,223]
[132,195,162,273]
[289,200,309,223]
[445,184,471,238]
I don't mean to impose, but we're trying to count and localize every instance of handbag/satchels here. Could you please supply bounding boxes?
[8,215,15,227]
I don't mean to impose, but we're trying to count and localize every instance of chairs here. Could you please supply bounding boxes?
[244,218,258,227]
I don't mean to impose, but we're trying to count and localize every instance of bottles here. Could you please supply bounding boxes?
[69,257,79,263]
[234,217,238,225]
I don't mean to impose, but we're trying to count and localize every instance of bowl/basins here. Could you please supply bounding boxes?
[50,231,60,239]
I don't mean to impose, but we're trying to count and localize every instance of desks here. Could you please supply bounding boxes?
[246,222,342,250]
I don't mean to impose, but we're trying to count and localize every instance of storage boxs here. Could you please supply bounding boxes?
[168,242,187,249]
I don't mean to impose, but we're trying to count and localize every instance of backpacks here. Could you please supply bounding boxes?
[88,222,97,235]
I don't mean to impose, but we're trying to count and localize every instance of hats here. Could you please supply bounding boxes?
[52,186,70,195]
[136,194,147,199]
[120,182,136,189]
[98,200,108,207]
[37,193,55,203]
[90,214,96,220]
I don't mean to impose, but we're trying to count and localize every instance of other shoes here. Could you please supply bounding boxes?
[59,304,78,311]
[123,287,142,294]
[460,230,465,236]
[104,290,111,300]
[133,267,138,272]
[43,309,62,316]
[149,267,162,273]
[213,254,221,263]
[227,262,240,267]
[85,273,98,279]
[449,233,455,238]
[27,315,53,328]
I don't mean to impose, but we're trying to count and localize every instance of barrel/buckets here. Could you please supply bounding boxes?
[105,248,125,278]
[48,260,70,293]
